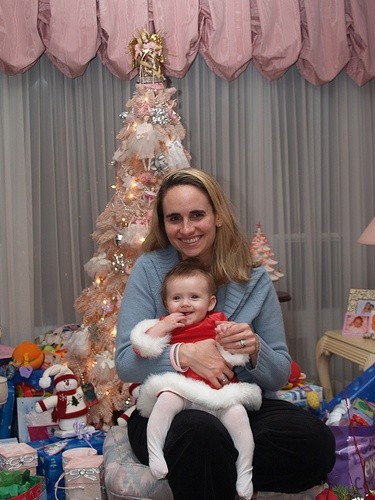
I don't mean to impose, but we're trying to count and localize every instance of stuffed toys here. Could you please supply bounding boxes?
[34,367,92,435]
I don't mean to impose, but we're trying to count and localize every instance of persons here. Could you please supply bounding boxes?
[358,302,375,316]
[350,317,366,332]
[128,258,258,500]
[114,166,336,499]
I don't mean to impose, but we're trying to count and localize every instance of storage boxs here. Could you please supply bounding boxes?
[0,382,107,500]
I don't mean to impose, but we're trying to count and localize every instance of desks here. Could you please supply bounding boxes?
[315,329,375,403]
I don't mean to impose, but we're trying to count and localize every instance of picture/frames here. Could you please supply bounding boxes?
[342,289,375,337]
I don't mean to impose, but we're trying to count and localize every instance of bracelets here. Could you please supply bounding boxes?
[170,342,191,373]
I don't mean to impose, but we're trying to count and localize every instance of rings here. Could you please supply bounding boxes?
[240,340,246,348]
[220,376,227,382]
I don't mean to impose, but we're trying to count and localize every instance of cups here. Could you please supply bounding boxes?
[61,447,98,471]
